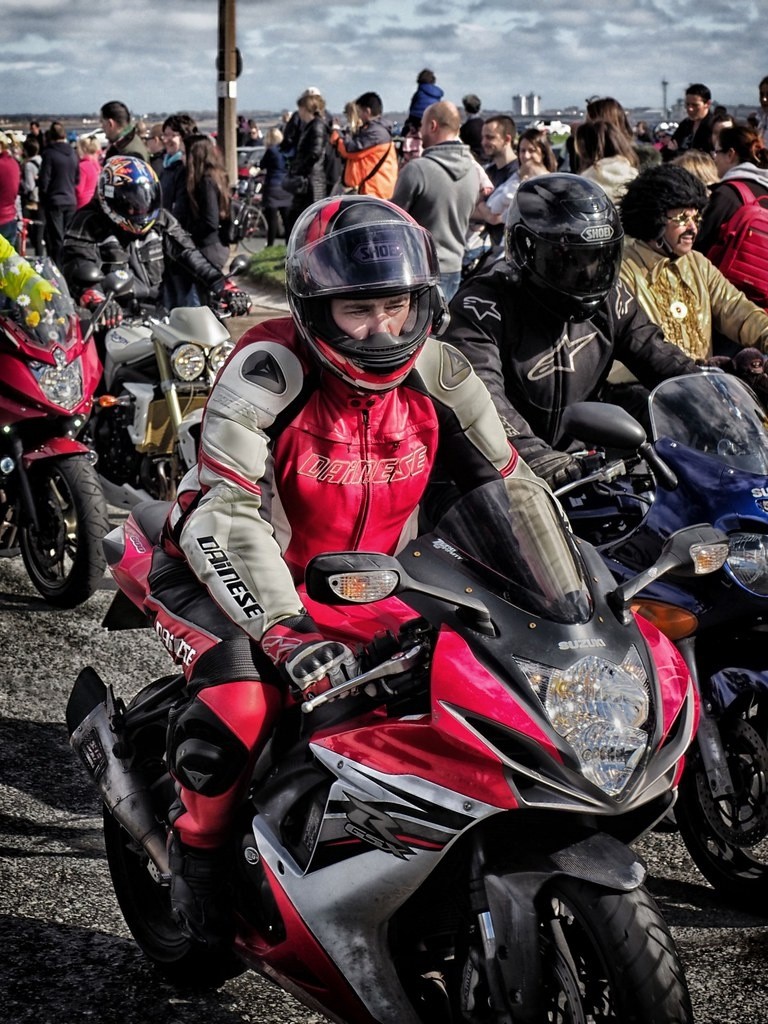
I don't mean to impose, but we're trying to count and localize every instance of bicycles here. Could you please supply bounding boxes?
[228,176,268,254]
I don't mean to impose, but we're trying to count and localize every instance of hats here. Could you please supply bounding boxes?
[653,121,679,137]
[297,87,321,104]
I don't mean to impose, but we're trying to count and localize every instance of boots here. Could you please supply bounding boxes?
[153,830,228,1008]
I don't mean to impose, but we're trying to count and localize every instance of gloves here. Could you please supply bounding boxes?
[505,434,584,491]
[259,614,364,707]
[210,276,253,317]
[78,289,122,329]
[700,408,751,452]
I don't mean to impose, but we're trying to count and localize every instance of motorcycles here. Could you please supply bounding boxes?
[0,255,135,612]
[550,371,768,922]
[64,476,734,1024]
[75,255,252,503]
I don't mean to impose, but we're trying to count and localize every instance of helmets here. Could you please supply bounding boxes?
[283,195,442,394]
[96,155,164,239]
[503,173,626,322]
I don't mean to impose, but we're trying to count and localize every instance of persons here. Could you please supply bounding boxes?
[0,100,263,435]
[146,195,546,943]
[258,69,768,339]
[421,164,768,529]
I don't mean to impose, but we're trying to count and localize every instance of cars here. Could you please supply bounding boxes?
[78,128,109,148]
[237,147,266,204]
[527,119,571,136]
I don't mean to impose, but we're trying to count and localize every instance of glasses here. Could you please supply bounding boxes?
[664,213,703,228]
[160,133,182,141]
[710,149,723,160]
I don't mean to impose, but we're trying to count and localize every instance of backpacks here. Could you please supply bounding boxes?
[219,197,256,243]
[706,181,768,313]
[324,124,343,183]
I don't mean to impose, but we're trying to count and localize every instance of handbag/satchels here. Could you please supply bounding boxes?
[329,175,360,196]
[280,174,309,194]
